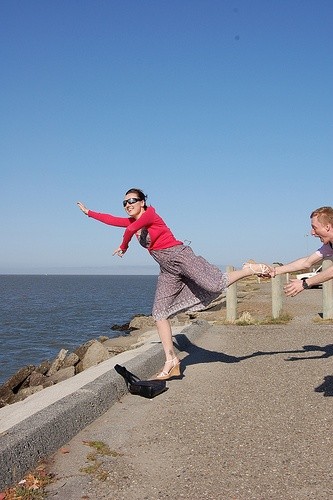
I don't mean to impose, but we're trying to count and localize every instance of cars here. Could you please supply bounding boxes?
[296,264,323,289]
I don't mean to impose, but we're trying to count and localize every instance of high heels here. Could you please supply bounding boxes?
[157,357,181,380]
[243,260,273,277]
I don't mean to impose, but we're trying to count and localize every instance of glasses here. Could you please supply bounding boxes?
[123,198,145,207]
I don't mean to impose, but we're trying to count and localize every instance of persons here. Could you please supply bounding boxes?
[267,207,333,296]
[76,188,274,379]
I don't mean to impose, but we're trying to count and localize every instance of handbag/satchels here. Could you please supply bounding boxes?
[114,363,166,398]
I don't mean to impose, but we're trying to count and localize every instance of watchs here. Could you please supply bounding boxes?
[302,279,309,289]
